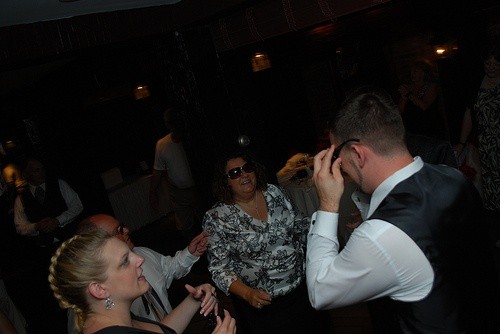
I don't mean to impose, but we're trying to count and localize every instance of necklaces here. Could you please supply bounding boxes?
[244,190,262,220]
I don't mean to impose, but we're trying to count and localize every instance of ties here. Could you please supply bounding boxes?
[34,187,45,199]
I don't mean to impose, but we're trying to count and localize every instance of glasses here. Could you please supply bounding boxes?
[226,164,255,180]
[331,139,361,161]
[112,223,124,237]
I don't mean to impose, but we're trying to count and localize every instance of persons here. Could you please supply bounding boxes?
[48,226,237,334]
[202,143,320,334]
[305,89,500,333]
[0,310,17,334]
[147,107,206,238]
[455,41,500,211]
[396,58,457,168]
[13,157,83,268]
[450,141,481,174]
[67,213,208,334]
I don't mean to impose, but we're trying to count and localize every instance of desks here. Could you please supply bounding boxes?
[276,153,358,229]
[108,169,177,233]
